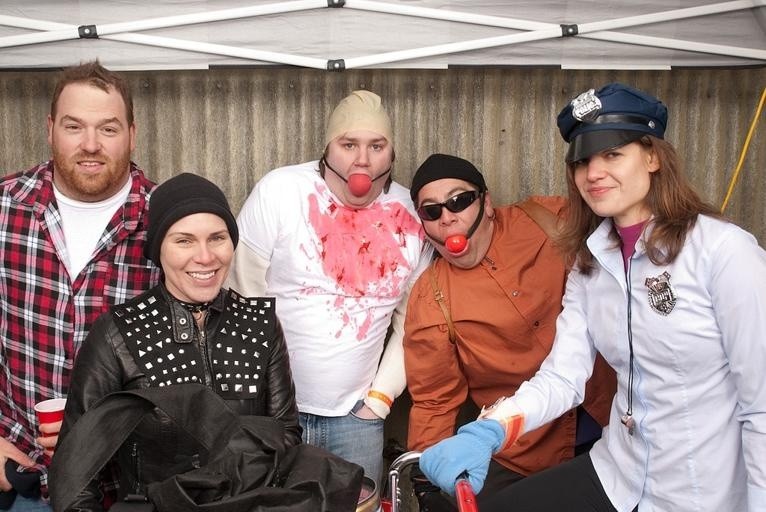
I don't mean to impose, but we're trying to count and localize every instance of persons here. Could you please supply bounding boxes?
[402,154,617,512]
[218,90,438,512]
[418,83,766,512]
[51,172,303,512]
[0,58,158,512]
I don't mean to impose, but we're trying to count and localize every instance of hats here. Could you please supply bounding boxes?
[322,87,395,156]
[410,154,489,201]
[556,82,670,166]
[142,171,240,271]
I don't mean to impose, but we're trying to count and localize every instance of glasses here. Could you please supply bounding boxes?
[416,190,482,221]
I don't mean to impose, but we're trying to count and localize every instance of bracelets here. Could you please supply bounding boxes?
[368,392,393,409]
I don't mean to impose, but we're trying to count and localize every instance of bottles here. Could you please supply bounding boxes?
[356,475,383,512]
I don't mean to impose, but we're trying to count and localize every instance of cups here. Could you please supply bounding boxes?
[35,399,67,455]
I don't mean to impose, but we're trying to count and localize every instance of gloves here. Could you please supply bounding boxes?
[418,415,507,500]
